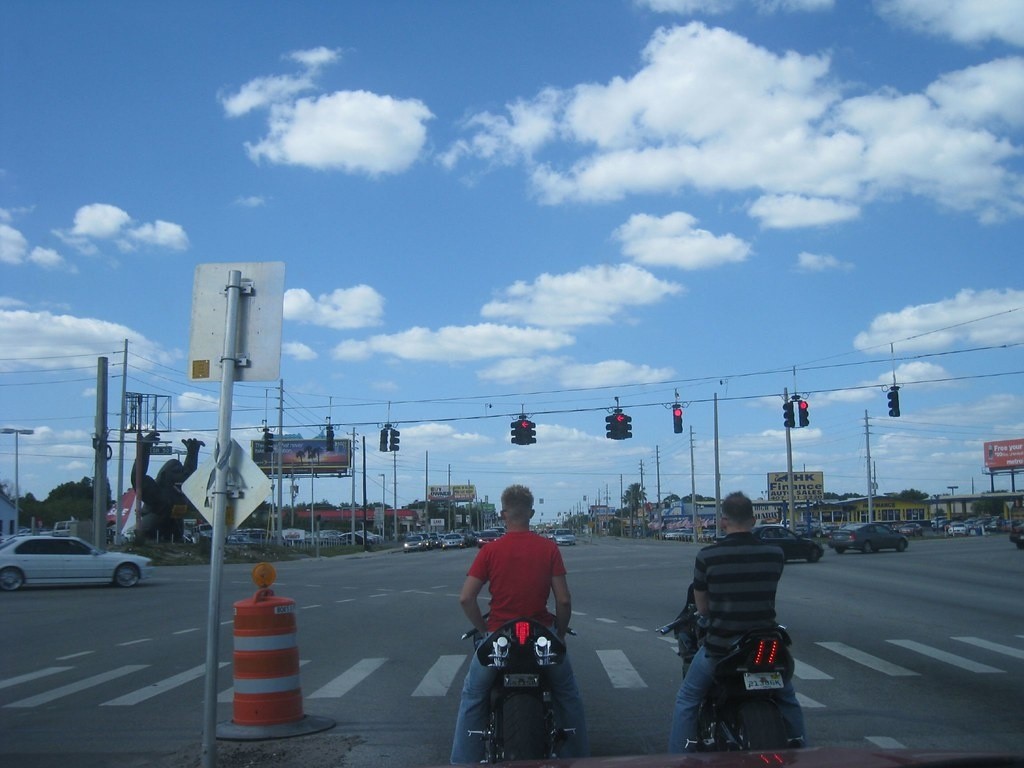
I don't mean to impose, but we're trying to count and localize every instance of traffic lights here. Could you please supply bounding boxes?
[264,432,274,452]
[326,430,335,451]
[798,401,810,427]
[380,430,388,452]
[782,401,795,428]
[389,428,400,451]
[887,391,900,417]
[673,408,683,433]
[605,413,632,440]
[510,420,537,445]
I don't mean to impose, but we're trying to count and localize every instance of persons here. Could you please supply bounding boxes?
[449,484,590,764]
[667,490,809,755]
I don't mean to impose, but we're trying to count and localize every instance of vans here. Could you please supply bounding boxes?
[54,520,75,537]
[185,524,212,543]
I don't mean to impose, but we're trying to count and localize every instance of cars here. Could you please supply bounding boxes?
[403,526,506,552]
[539,528,576,545]
[226,528,384,547]
[931,515,1024,550]
[828,519,924,553]
[665,527,726,540]
[0,528,155,592]
[779,518,839,538]
[752,525,824,560]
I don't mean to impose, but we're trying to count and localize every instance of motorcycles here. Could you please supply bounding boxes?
[462,613,580,763]
[655,581,807,765]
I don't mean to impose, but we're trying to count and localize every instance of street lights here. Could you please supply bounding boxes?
[0,428,34,537]
[947,486,959,496]
[378,474,385,519]
